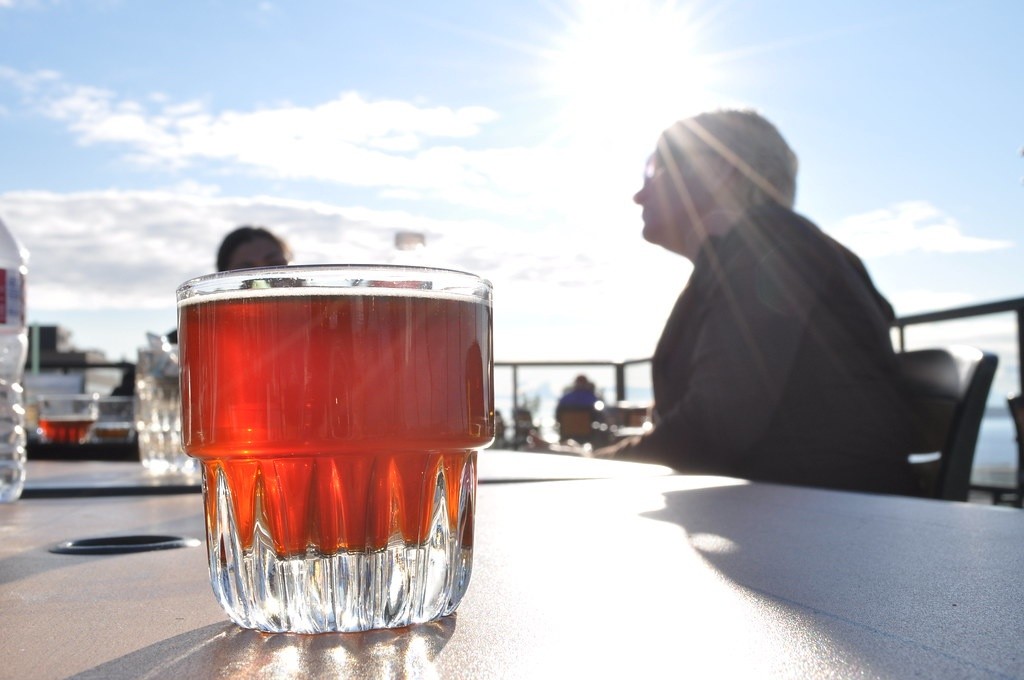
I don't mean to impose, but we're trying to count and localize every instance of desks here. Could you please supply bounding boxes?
[0,449,1024,680]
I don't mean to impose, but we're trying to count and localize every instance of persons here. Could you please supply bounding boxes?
[556,376,599,445]
[217,228,288,272]
[589,110,921,496]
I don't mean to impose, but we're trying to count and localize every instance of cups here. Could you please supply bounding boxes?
[174,263,497,635]
[135,345,204,486]
[92,397,138,442]
[40,394,99,442]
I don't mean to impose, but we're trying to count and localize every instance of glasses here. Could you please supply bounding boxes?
[643,151,735,183]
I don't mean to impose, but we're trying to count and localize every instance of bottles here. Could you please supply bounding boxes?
[1,219,30,502]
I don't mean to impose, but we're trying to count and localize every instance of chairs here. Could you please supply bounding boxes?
[894,348,998,502]
